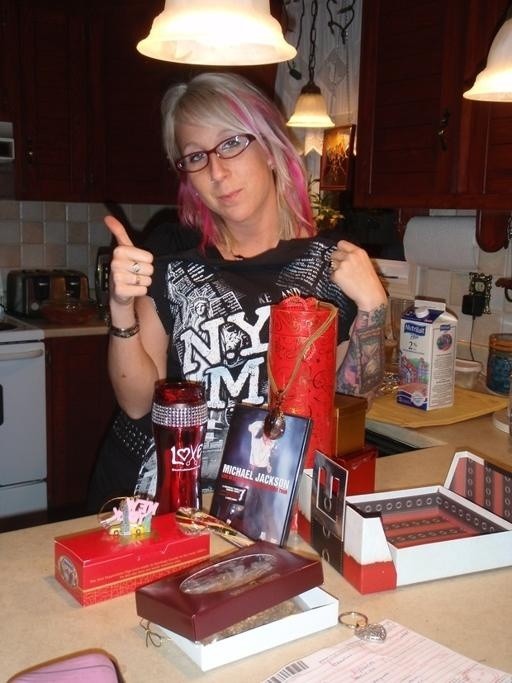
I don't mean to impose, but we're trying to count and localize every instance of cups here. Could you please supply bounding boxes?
[391,299,415,341]
[151,378,208,513]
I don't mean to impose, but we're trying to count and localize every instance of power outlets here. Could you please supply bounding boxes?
[469,272,492,315]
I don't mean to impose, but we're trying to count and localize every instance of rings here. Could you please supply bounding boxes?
[132,261,141,274]
[136,274,140,285]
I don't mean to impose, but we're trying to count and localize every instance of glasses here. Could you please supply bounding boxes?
[175,133,258,173]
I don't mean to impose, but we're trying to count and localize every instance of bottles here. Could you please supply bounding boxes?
[485,332,512,398]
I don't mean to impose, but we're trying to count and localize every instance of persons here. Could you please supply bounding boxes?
[87,71,391,517]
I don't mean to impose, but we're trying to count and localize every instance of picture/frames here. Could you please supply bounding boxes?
[319,124,356,190]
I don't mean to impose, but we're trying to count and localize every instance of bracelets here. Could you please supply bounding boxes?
[107,320,141,339]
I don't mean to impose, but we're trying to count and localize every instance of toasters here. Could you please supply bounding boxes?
[7,269,90,318]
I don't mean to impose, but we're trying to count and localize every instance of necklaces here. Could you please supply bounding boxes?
[264,297,337,440]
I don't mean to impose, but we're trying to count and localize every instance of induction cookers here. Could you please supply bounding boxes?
[0,314,46,343]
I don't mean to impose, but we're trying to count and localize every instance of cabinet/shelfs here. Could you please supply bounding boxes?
[47,337,121,512]
[0,0,186,205]
[352,0,512,211]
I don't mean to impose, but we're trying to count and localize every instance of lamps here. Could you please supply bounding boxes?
[463,19,512,103]
[135,0,298,66]
[285,0,335,129]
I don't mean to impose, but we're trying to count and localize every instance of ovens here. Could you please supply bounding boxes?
[0,342,48,520]
